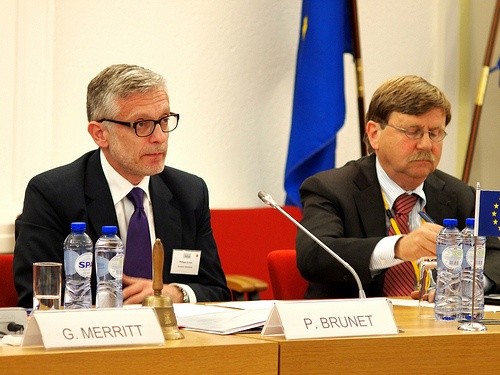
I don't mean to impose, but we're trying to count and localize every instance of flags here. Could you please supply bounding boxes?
[283,0,353,208]
[479,190,500,236]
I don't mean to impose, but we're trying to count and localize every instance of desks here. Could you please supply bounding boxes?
[0,296,500,375]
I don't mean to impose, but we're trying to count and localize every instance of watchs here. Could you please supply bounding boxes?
[174,285,189,302]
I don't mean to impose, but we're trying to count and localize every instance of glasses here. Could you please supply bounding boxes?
[382,122,448,142]
[96,112,180,137]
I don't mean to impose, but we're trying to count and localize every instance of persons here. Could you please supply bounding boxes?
[12,64,233,306]
[296,76,500,304]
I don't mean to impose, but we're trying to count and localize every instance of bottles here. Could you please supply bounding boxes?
[94,226,124,308]
[434,218,463,320]
[460,218,489,321]
[63,222,93,309]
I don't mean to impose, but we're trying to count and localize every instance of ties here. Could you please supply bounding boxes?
[383,193,418,297]
[122,187,152,290]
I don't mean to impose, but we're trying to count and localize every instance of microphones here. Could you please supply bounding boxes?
[256,192,366,300]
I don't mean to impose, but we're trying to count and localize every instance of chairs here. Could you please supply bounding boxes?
[208,208,312,301]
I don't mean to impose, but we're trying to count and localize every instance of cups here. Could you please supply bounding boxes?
[33,261,62,309]
[417,255,438,319]
